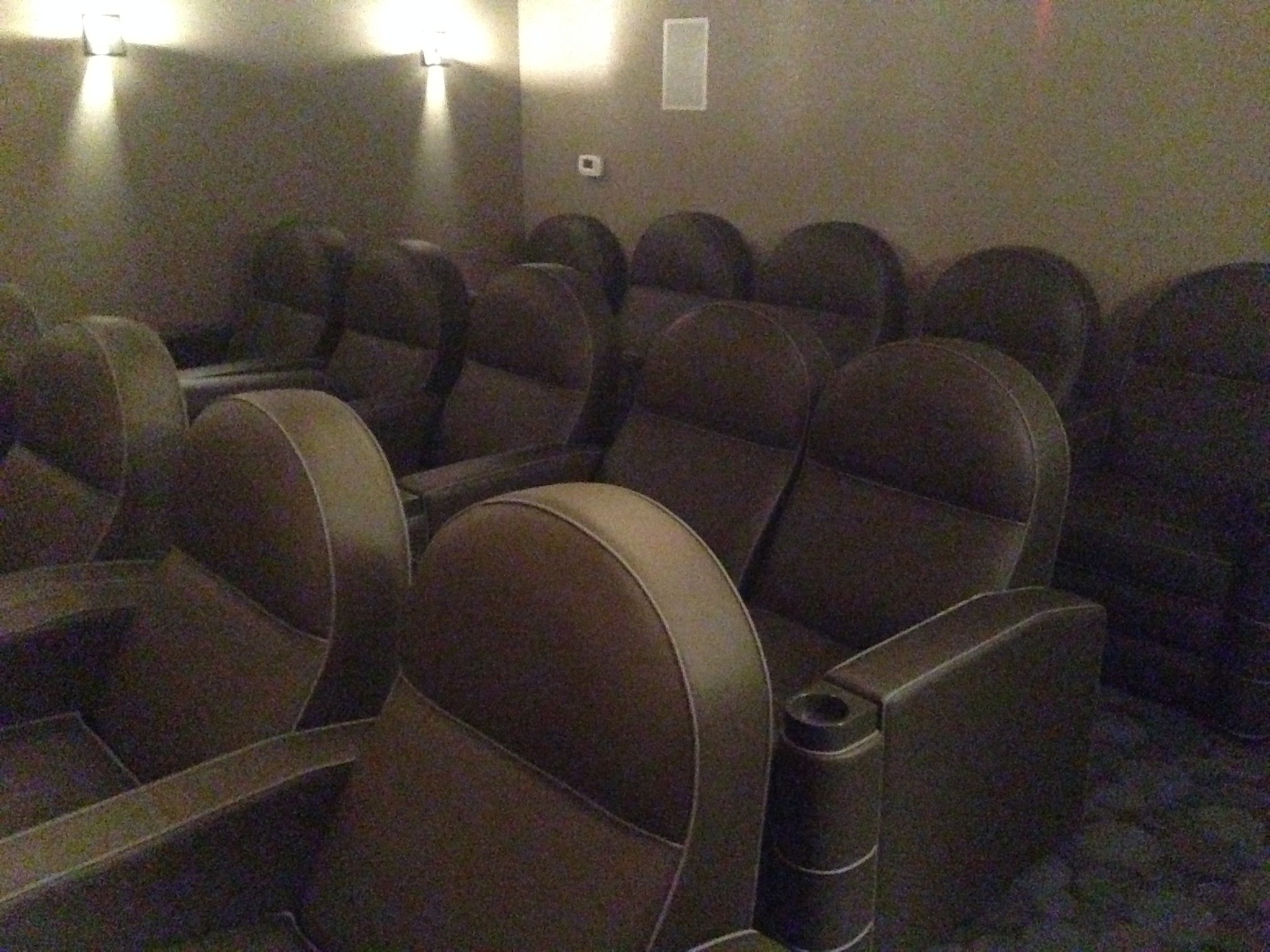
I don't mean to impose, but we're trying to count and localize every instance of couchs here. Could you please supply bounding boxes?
[513,216,628,316]
[181,241,470,419]
[1067,263,1270,739]
[343,263,621,555]
[0,315,190,577]
[600,298,1109,952]
[753,223,909,358]
[0,281,49,470]
[0,480,786,952]
[0,385,414,840]
[142,200,356,371]
[616,211,755,366]
[899,245,1106,420]
